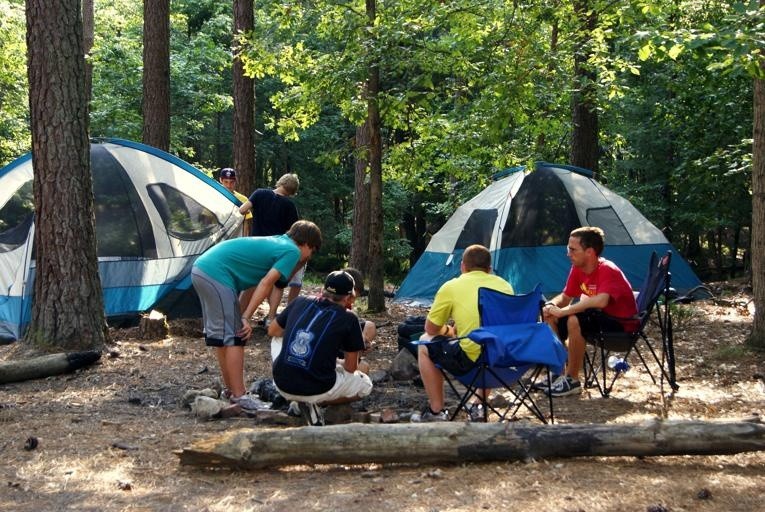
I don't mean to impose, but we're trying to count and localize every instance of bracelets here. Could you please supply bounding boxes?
[445,326,449,335]
[544,302,555,306]
[241,317,249,321]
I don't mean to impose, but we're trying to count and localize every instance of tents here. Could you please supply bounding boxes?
[0,137,245,344]
[394,162,714,307]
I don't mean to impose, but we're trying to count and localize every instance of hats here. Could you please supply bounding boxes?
[324,271,356,296]
[220,167,237,180]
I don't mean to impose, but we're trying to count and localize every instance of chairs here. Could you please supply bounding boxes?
[583,248,680,399]
[411,282,568,425]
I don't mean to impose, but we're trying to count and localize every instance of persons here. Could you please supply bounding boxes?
[201,167,253,241]
[339,268,376,375]
[533,226,642,396]
[287,263,308,303]
[268,271,373,426]
[191,220,323,412]
[239,173,299,329]
[410,245,514,422]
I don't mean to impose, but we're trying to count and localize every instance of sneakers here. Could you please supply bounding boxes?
[410,406,448,423]
[287,402,325,427]
[545,374,584,398]
[470,403,489,422]
[263,316,271,329]
[535,374,560,389]
[220,389,274,416]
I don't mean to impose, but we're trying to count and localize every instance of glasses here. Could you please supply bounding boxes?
[567,245,583,253]
